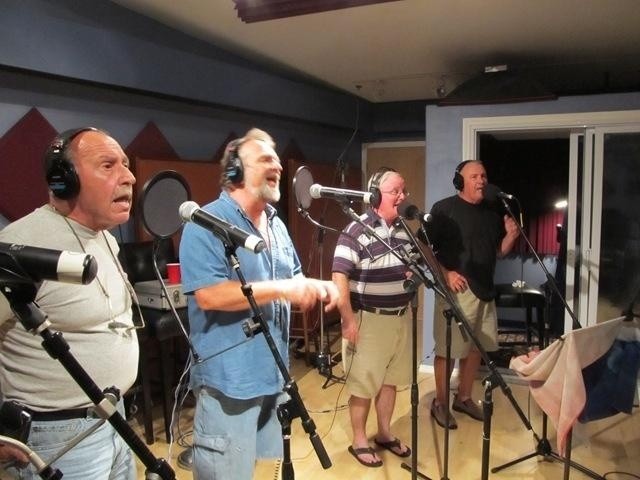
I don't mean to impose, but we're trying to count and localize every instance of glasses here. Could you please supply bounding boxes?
[382,190,410,197]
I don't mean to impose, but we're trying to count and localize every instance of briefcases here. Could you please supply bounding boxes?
[133,280,188,312]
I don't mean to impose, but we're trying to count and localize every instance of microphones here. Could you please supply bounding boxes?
[310,184,372,204]
[482,184,513,203]
[178,201,265,254]
[0,241,98,285]
[398,202,433,223]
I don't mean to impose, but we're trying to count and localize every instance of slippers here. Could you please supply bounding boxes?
[374,436,411,457]
[348,446,383,467]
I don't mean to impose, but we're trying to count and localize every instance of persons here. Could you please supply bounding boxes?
[415,157,523,431]
[0,123,144,480]
[175,125,345,480]
[330,166,425,469]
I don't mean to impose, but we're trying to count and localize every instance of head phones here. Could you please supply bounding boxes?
[45,127,98,200]
[225,138,243,184]
[453,160,474,191]
[369,167,396,208]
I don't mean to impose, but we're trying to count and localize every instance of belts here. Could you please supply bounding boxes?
[31,408,96,422]
[359,306,407,315]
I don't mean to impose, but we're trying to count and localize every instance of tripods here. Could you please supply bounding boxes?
[320,223,346,389]
[491,203,605,480]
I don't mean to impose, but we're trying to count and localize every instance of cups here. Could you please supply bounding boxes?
[166,263,183,285]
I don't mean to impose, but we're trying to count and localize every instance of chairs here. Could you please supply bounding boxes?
[118,237,190,443]
[494,282,545,350]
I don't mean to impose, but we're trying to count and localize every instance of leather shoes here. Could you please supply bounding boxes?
[452,393,484,421]
[431,398,457,429]
